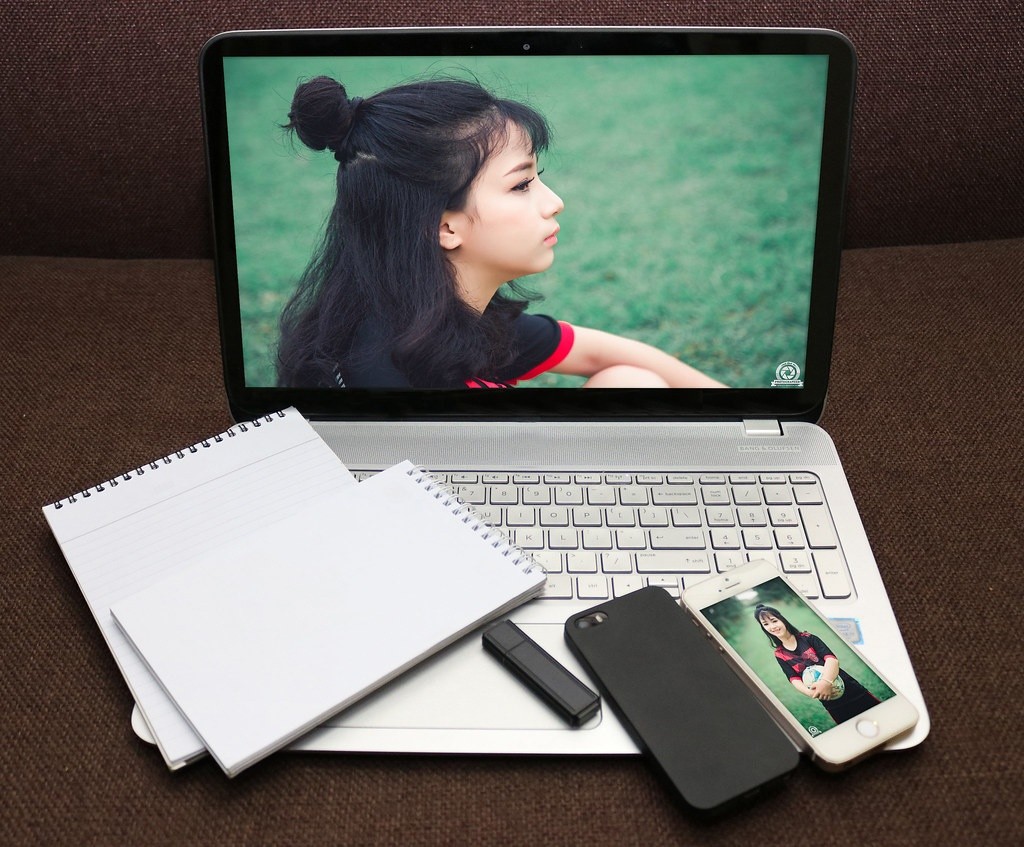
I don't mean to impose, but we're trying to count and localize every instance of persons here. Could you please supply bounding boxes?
[276,75,730,388]
[754,604,881,725]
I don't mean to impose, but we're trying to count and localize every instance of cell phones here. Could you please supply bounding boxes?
[565,586,801,812]
[680,558,921,771]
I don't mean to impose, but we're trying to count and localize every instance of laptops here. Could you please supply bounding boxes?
[199,28,929,757]
